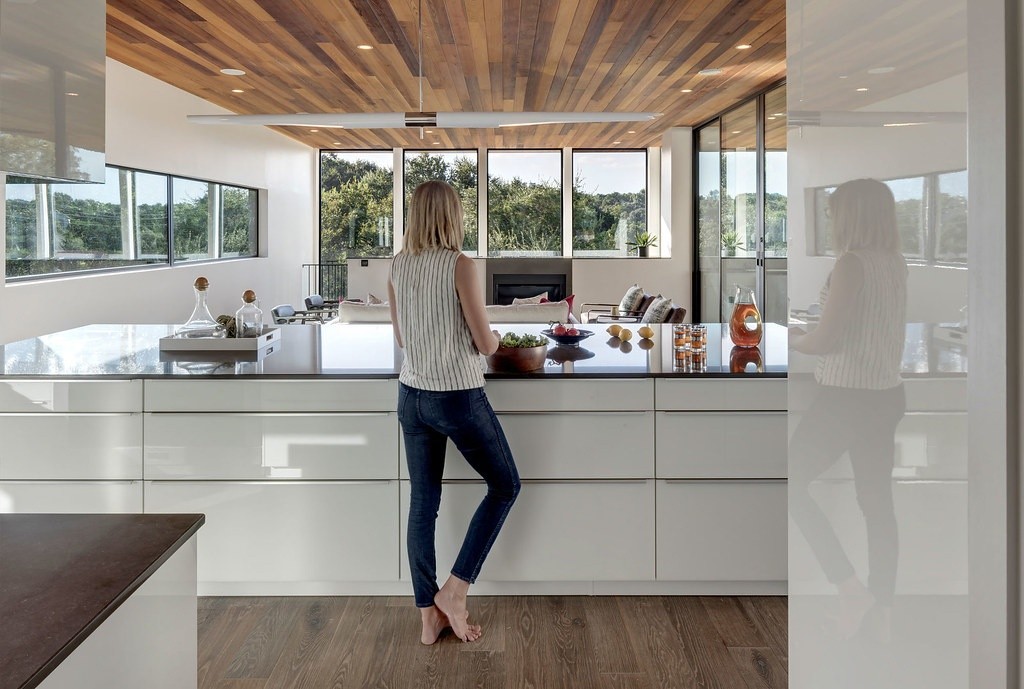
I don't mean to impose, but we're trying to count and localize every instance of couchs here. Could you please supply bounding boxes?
[338,296,581,324]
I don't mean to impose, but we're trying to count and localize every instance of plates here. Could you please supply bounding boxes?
[541,329,594,345]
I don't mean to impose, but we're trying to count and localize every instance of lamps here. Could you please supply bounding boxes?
[186,0,666,139]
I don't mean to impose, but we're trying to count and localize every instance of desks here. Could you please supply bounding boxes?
[0,513,205,689]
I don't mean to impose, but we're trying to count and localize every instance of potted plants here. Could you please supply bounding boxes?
[722,232,748,256]
[625,231,658,257]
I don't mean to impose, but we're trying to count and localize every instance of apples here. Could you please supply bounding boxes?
[552,324,580,335]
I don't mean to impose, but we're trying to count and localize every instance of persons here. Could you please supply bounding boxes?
[789,178,909,638]
[386,181,522,645]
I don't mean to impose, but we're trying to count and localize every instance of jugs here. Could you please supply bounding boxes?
[729,285,763,346]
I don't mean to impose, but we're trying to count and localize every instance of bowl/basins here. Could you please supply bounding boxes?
[484,340,547,371]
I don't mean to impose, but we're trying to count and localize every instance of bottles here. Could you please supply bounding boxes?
[236,298,263,337]
[174,285,228,338]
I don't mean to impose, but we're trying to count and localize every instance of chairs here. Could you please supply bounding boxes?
[580,293,656,323]
[305,295,339,320]
[271,304,336,325]
[595,302,687,323]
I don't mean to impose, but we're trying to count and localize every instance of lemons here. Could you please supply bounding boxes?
[606,325,623,336]
[618,329,632,342]
[637,327,654,338]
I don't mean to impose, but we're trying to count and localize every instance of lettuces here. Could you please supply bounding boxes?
[500,332,550,348]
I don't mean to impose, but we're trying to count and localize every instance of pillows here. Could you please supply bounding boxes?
[618,284,644,316]
[512,292,548,305]
[640,292,673,323]
[540,295,575,324]
